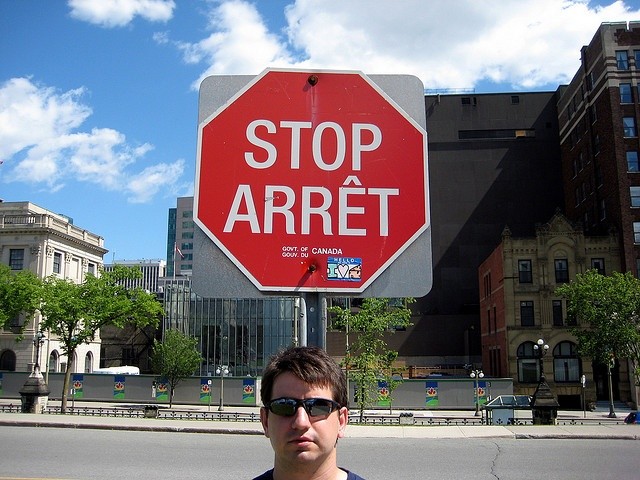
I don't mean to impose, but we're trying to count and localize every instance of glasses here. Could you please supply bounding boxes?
[265,397,342,418]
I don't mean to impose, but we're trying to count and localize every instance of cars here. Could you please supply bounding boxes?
[624,412,640,425]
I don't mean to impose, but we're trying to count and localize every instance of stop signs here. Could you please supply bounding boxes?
[194,67,431,294]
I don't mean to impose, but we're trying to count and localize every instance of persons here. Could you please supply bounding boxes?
[247,343,365,480]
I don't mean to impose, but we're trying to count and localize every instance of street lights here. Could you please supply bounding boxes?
[533,338,550,380]
[32,332,45,374]
[214,364,230,411]
[470,370,485,417]
